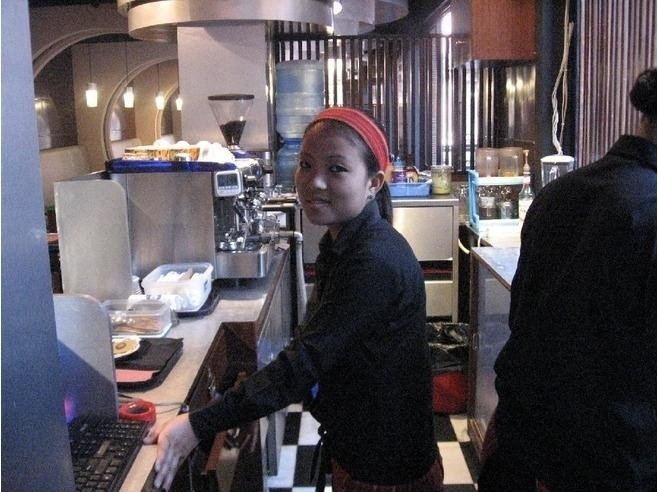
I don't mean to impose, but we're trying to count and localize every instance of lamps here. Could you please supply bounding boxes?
[84,34,98,108]
[176,61,183,111]
[153,64,165,108]
[121,37,134,109]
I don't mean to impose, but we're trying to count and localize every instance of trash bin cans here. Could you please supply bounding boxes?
[424,321,470,414]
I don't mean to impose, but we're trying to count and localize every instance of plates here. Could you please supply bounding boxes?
[110,335,139,360]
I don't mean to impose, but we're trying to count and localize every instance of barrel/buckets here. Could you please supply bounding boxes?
[275,59,326,140]
[276,139,302,188]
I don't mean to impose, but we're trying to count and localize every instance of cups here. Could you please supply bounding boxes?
[122,146,201,162]
[540,155,575,190]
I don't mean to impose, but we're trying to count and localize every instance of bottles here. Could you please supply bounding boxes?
[276,138,304,193]
[390,154,418,184]
[475,147,536,227]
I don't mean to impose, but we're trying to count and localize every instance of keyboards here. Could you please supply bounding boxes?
[67,415,151,492]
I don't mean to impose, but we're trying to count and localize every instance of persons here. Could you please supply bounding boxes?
[142,107,445,492]
[477,66,657,492]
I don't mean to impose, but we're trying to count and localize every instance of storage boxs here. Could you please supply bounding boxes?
[142,263,214,312]
